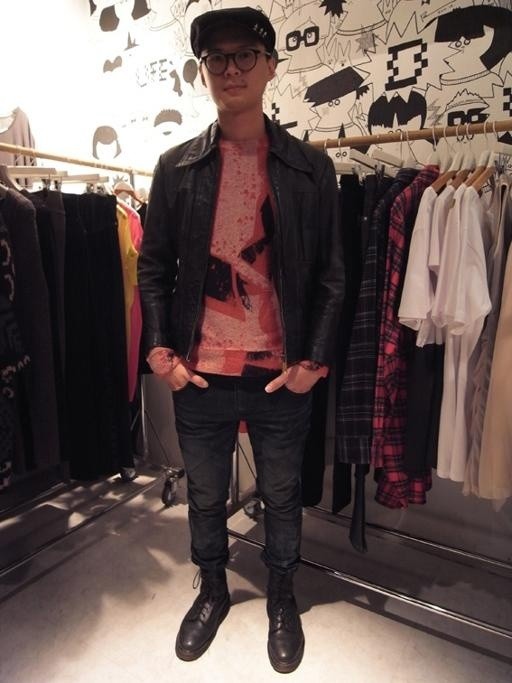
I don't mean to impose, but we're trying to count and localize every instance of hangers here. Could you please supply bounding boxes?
[429,119,499,208]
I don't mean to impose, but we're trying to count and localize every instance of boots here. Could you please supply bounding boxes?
[176,565,229,661]
[267,569,304,672]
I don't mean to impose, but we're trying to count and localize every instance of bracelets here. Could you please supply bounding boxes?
[299,362,323,371]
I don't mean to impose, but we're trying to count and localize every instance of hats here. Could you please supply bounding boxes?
[191,8,275,59]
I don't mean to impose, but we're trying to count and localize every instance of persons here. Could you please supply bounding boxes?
[136,7,347,672]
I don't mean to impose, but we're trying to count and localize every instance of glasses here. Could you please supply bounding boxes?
[448,108,486,125]
[286,26,318,51]
[200,48,271,75]
